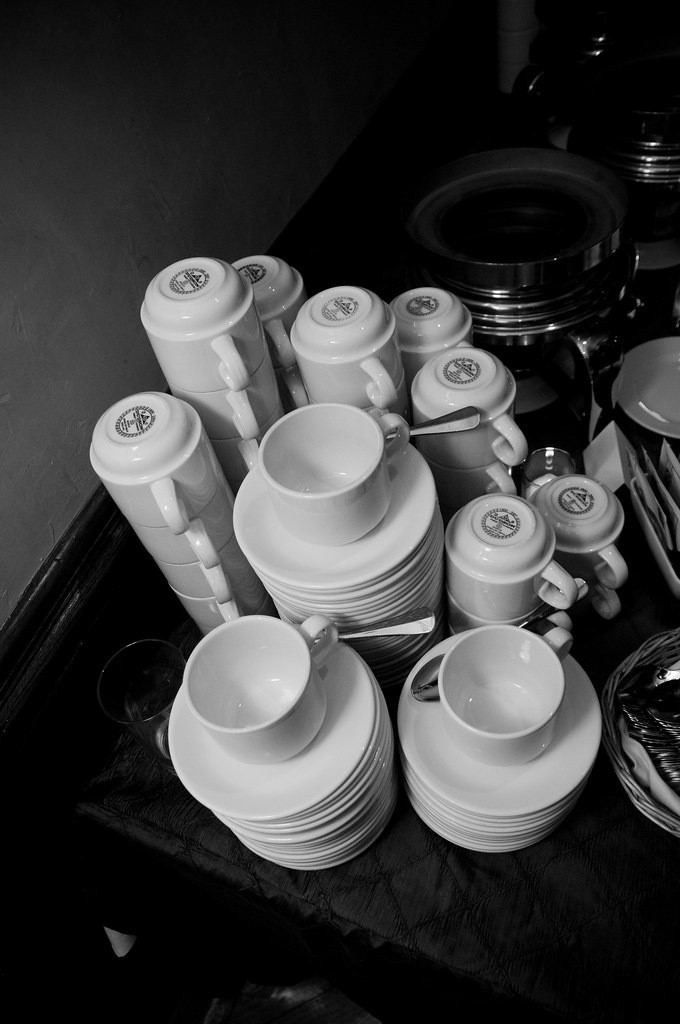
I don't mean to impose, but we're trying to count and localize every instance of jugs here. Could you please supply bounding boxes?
[397,145,641,465]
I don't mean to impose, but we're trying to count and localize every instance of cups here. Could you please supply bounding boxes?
[436,626,575,768]
[181,613,339,767]
[520,447,577,507]
[388,287,475,393]
[96,638,186,782]
[228,255,309,415]
[89,390,274,640]
[140,256,284,494]
[257,402,410,549]
[527,475,630,621]
[410,347,529,510]
[445,492,579,641]
[290,286,410,428]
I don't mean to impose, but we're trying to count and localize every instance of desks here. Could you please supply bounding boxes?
[71,610,680,1024]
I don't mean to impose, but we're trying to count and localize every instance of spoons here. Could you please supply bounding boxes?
[410,601,555,703]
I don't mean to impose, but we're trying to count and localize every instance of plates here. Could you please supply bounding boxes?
[168,639,395,872]
[398,625,603,854]
[614,336,680,440]
[226,439,446,702]
